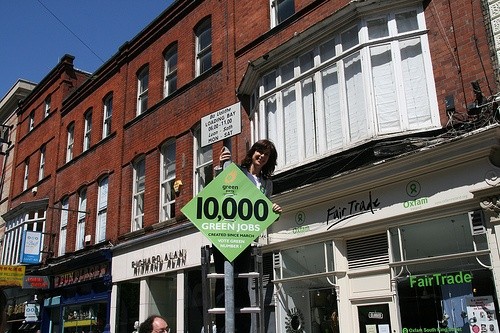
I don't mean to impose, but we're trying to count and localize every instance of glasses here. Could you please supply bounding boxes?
[153,328,170,333]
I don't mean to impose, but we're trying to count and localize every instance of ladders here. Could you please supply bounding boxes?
[201,245,266,333]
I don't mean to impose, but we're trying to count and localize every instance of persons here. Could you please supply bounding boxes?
[216,138,284,333]
[137,314,172,333]
